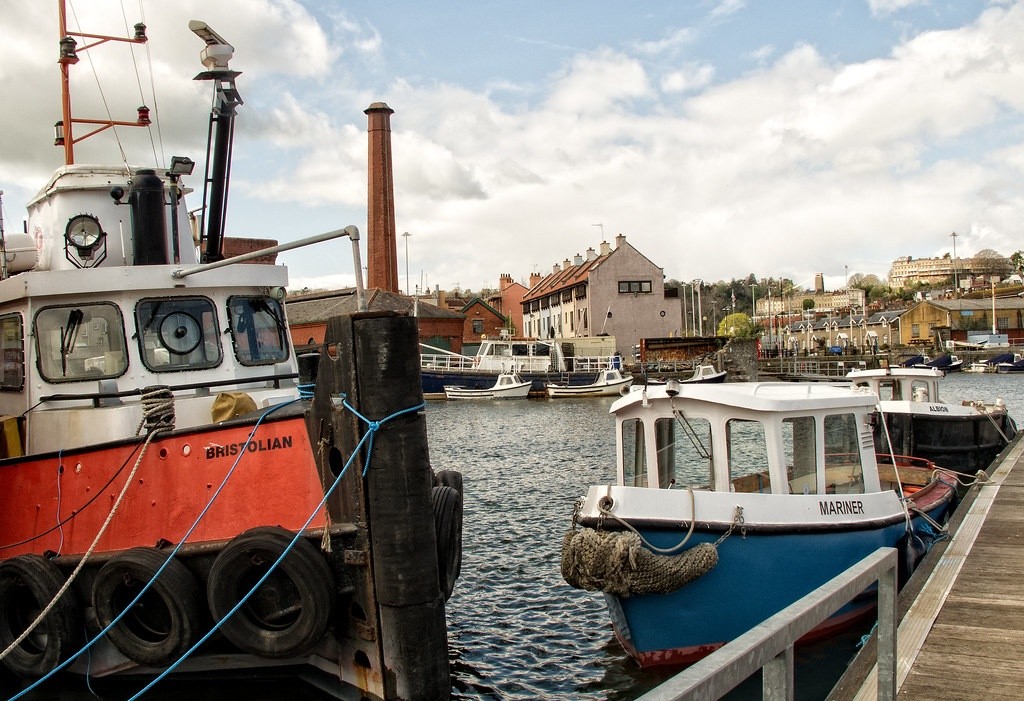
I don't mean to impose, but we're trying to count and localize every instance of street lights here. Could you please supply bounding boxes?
[950,232,959,299]
[591,224,603,242]
[749,283,758,327]
[842,265,850,311]
[768,286,773,347]
[711,301,716,336]
[703,317,708,336]
[722,308,730,336]
[402,232,412,297]
[680,282,688,337]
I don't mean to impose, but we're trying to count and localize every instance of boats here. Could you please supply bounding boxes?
[0,17,339,701]
[444,374,533,401]
[847,365,1009,472]
[421,339,600,399]
[545,368,633,396]
[647,365,726,385]
[899,353,1024,373]
[576,377,959,670]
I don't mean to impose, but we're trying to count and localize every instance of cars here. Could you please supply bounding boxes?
[827,347,841,354]
